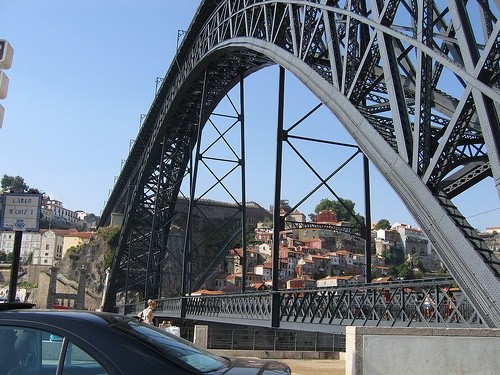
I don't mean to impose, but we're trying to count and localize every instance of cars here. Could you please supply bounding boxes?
[0,302,291,375]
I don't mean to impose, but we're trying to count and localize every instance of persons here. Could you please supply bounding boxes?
[162,320,180,338]
[138,299,157,327]
[382,290,392,321]
[258,281,267,290]
[412,288,461,324]
